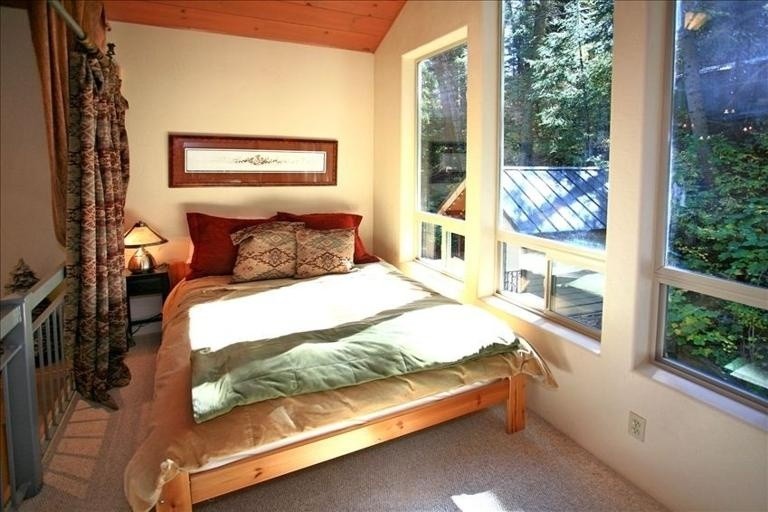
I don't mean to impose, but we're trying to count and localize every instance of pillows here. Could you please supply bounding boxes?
[183,208,379,282]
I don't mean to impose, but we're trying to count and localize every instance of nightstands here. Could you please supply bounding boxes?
[123,264,172,346]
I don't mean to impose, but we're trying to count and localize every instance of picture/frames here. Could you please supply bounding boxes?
[168,132,338,189]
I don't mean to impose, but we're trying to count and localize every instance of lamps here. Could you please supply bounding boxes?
[122,222,168,273]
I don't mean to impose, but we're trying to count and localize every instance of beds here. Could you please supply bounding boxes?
[155,260,534,512]
